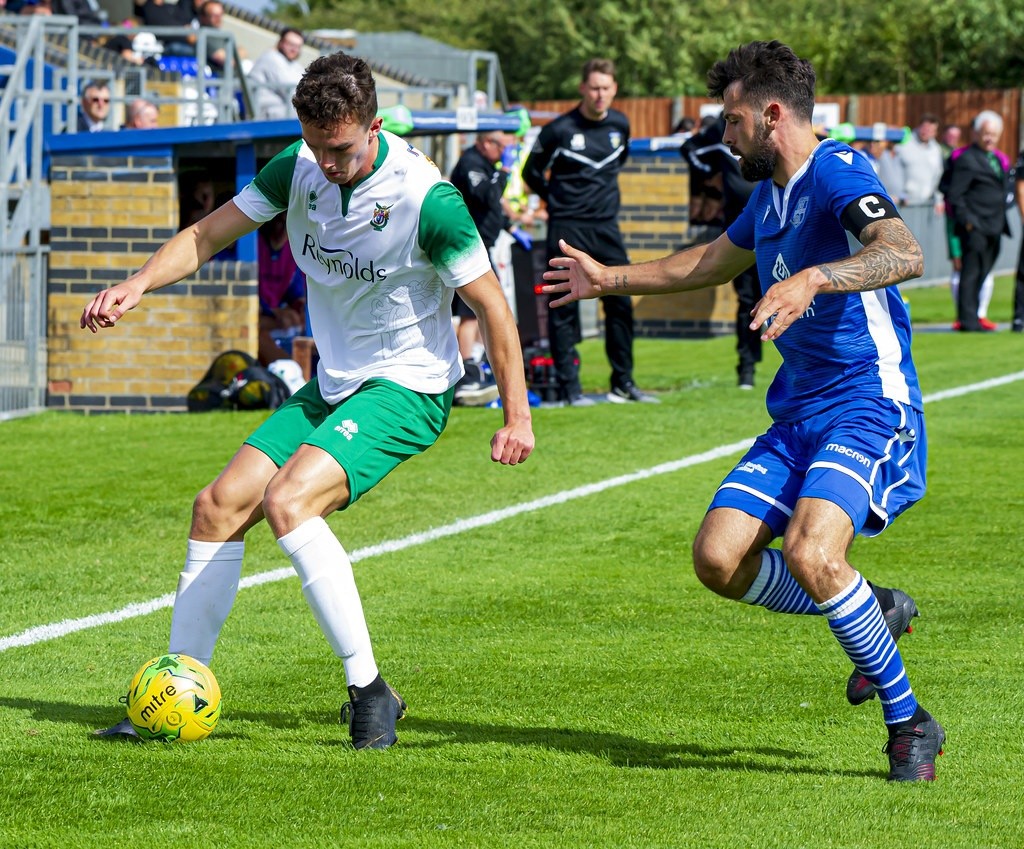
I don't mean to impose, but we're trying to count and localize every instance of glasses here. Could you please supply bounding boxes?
[92,98,110,104]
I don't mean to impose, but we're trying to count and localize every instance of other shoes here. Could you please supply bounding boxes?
[735,364,755,390]
[1012,317,1024,332]
[952,321,960,331]
[959,326,993,332]
[978,318,995,329]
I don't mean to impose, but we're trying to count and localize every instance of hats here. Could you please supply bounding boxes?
[132,33,164,53]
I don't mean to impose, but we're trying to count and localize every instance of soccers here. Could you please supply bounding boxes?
[126,653,222,743]
[186,350,308,413]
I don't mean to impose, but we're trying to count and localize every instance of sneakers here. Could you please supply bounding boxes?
[455,377,500,406]
[91,717,141,740]
[606,380,662,404]
[340,674,408,751]
[556,394,596,408]
[881,707,945,783]
[846,588,920,706]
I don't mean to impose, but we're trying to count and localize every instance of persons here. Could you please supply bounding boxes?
[0,0,305,135]
[521,58,661,407]
[80,50,551,752]
[543,40,1024,781]
